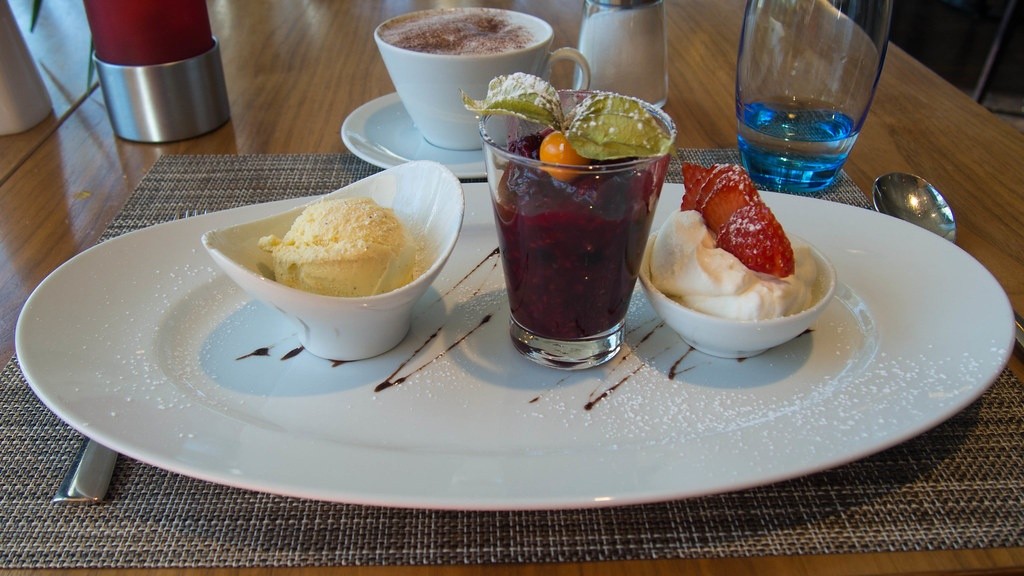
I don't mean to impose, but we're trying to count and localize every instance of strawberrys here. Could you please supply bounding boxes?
[680,160,796,277]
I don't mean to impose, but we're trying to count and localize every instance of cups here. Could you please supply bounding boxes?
[734,0,892,194]
[373,6,591,151]
[476,90,678,372]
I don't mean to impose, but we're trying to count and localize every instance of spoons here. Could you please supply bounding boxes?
[872,172,1024,351]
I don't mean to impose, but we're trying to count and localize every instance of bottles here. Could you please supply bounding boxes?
[573,0,669,111]
[81,0,214,66]
[0,0,53,137]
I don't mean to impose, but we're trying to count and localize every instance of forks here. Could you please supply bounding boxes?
[49,207,210,506]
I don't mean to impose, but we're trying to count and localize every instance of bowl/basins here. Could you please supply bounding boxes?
[635,230,837,360]
[199,159,466,362]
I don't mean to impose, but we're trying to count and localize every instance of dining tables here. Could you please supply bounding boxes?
[0,0,1024,576]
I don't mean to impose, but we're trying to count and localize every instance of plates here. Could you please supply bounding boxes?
[340,92,489,180]
[15,182,1016,511]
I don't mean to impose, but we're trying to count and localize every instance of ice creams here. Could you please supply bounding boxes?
[266,194,416,297]
